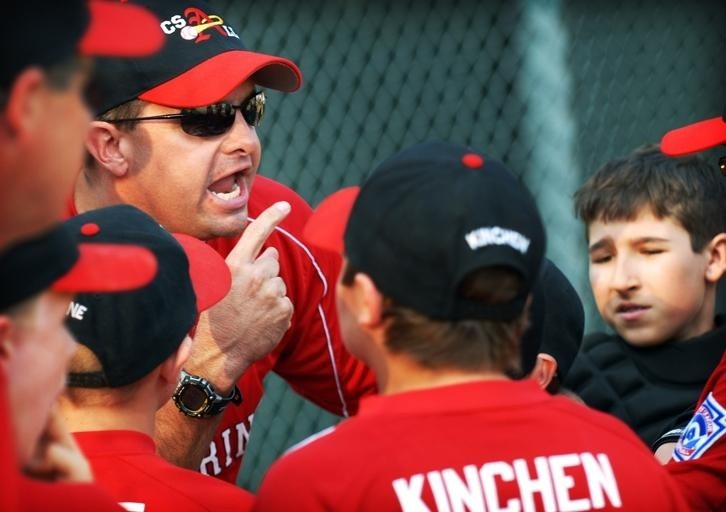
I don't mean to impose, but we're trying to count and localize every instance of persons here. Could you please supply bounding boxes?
[251,150,691,512]
[2,0,586,512]
[563,145,726,512]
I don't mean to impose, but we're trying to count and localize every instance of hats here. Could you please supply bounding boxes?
[60,203,234,388]
[496,252,589,393]
[0,0,171,120]
[302,137,547,325]
[81,0,305,112]
[0,221,161,314]
[657,103,726,179]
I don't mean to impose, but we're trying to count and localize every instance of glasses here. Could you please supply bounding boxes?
[93,88,272,140]
